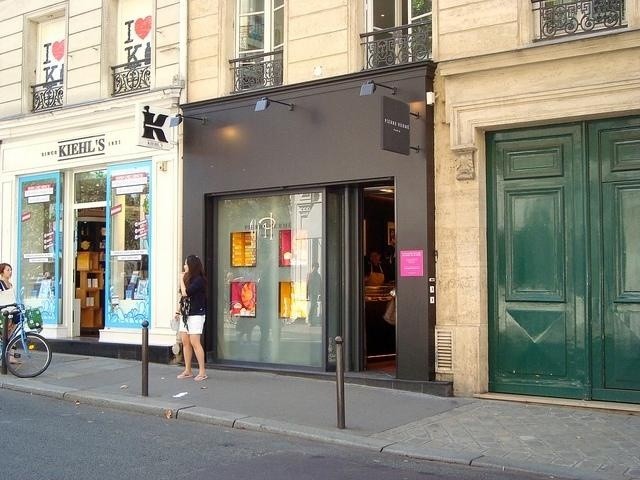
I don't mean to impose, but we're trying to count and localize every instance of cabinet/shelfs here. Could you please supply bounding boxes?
[75,251,105,332]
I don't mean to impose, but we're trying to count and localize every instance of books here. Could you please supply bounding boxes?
[86,278,99,306]
[125,271,150,301]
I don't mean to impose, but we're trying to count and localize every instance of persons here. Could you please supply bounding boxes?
[0,262,19,364]
[305,263,323,326]
[363,250,391,285]
[174,255,209,382]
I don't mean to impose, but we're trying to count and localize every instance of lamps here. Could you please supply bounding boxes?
[168,114,206,127]
[254,96,295,113]
[360,80,398,96]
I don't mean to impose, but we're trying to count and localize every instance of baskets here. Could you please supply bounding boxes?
[26,308,43,328]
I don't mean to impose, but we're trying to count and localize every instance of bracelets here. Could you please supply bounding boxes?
[176,312,181,315]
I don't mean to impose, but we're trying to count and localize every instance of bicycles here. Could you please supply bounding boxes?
[0,303,52,378]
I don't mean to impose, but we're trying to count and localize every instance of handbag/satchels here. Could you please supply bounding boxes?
[383,298,396,324]
[0,287,16,307]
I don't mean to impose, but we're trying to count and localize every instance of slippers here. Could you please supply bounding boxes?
[177,372,207,381]
